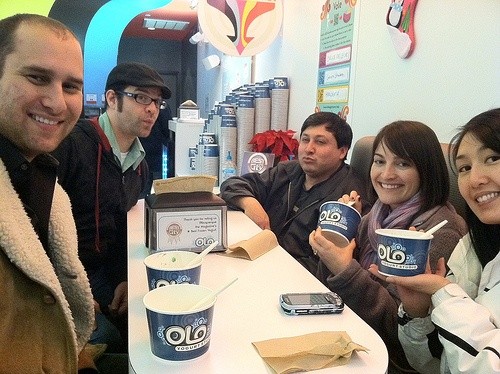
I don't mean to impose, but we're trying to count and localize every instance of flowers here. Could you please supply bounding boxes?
[248,128,300,161]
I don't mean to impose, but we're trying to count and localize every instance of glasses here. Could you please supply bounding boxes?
[118,91,166,109]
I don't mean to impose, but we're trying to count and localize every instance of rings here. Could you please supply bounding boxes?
[314,249,318,254]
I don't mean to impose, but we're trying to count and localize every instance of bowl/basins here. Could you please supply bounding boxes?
[188,76,289,187]
[143,284,217,361]
[143,250,204,291]
[374,228,435,278]
[319,201,361,248]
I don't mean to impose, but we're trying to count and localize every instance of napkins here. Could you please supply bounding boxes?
[251,330,371,374]
[225,229,279,261]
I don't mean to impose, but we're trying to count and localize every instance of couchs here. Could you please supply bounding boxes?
[349,137,469,238]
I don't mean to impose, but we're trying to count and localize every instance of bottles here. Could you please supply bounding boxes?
[221,151,237,184]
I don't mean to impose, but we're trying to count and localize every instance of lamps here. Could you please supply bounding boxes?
[189,32,202,45]
[201,54,220,70]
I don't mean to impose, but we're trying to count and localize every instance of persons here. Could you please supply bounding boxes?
[219,112,376,280]
[369,108,500,374]
[309,121,468,374]
[51,62,171,354]
[0,14,130,374]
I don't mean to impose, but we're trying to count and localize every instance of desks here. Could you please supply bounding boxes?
[168,117,207,178]
[126,197,388,374]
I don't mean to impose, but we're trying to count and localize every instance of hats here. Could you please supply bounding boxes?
[106,62,171,99]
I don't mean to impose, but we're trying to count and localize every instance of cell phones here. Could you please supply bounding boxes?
[280,292,345,315]
